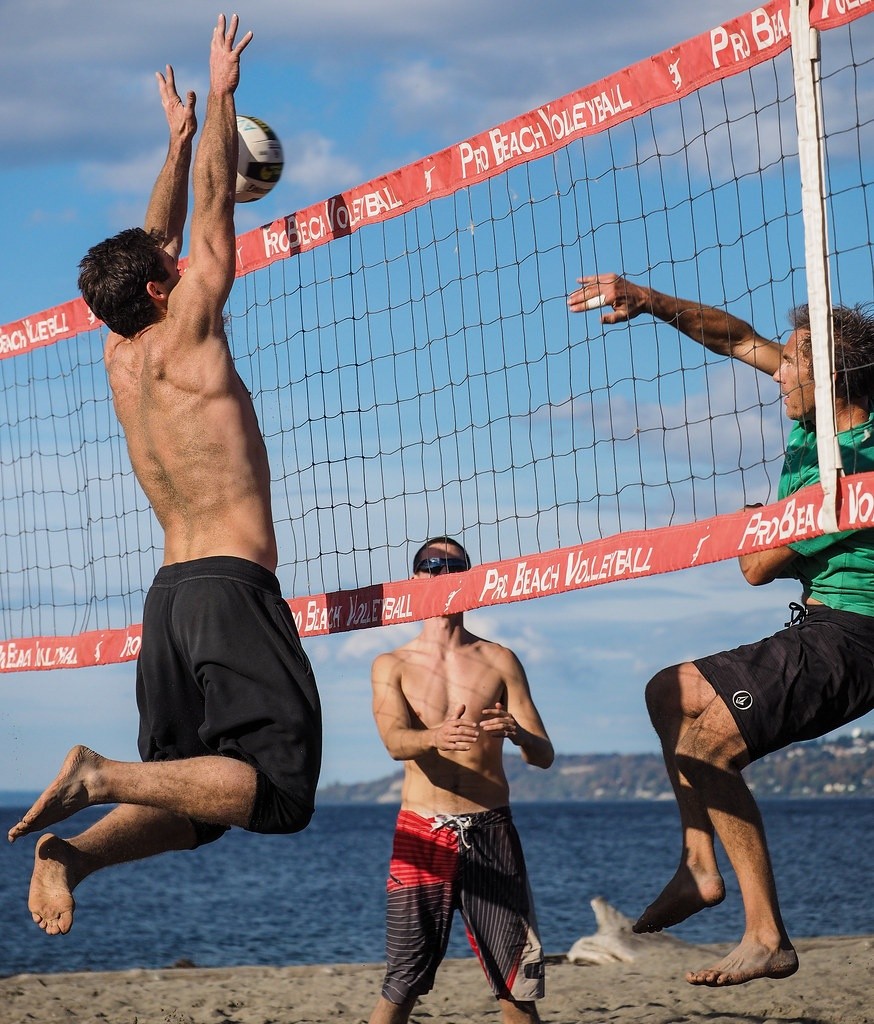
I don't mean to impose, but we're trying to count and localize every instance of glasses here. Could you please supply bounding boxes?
[416,557,466,574]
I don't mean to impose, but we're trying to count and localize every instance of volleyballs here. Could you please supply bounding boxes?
[230,115,285,204]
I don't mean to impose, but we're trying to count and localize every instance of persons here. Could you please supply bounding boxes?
[8,15,321,935]
[370,535,553,1023]
[568,275,874,987]
